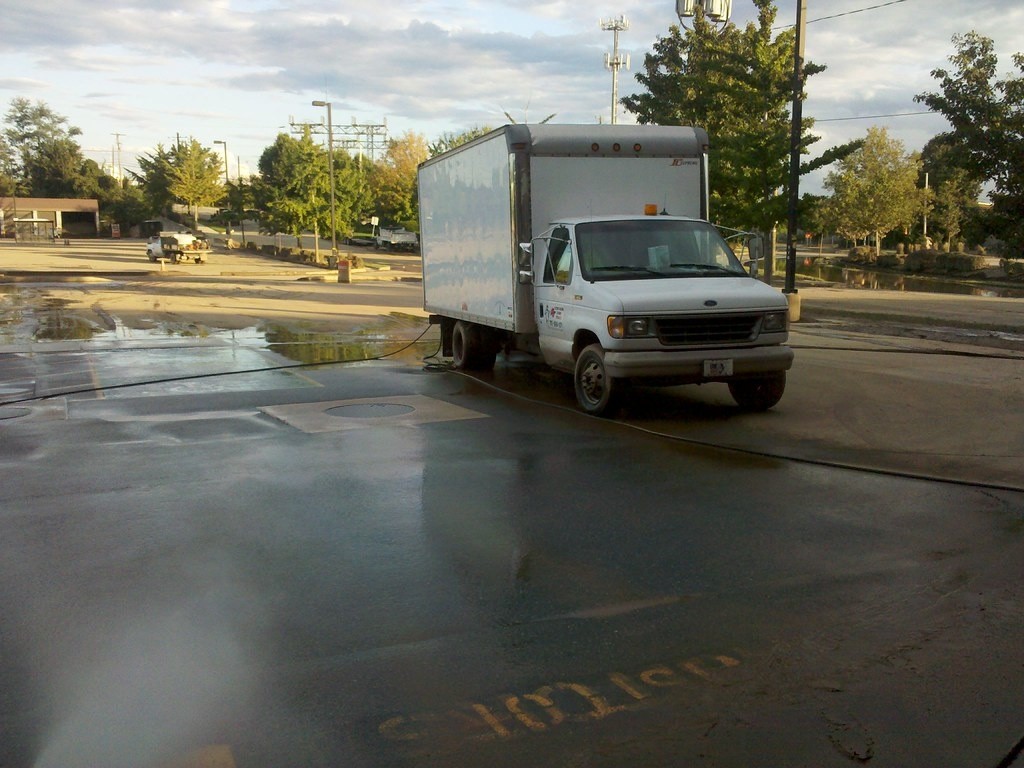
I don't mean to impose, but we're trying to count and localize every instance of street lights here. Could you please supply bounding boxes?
[214,140,231,227]
[313,101,336,257]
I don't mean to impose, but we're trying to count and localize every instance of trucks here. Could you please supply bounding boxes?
[375,227,419,252]
[416,124,794,413]
[146,232,213,265]
